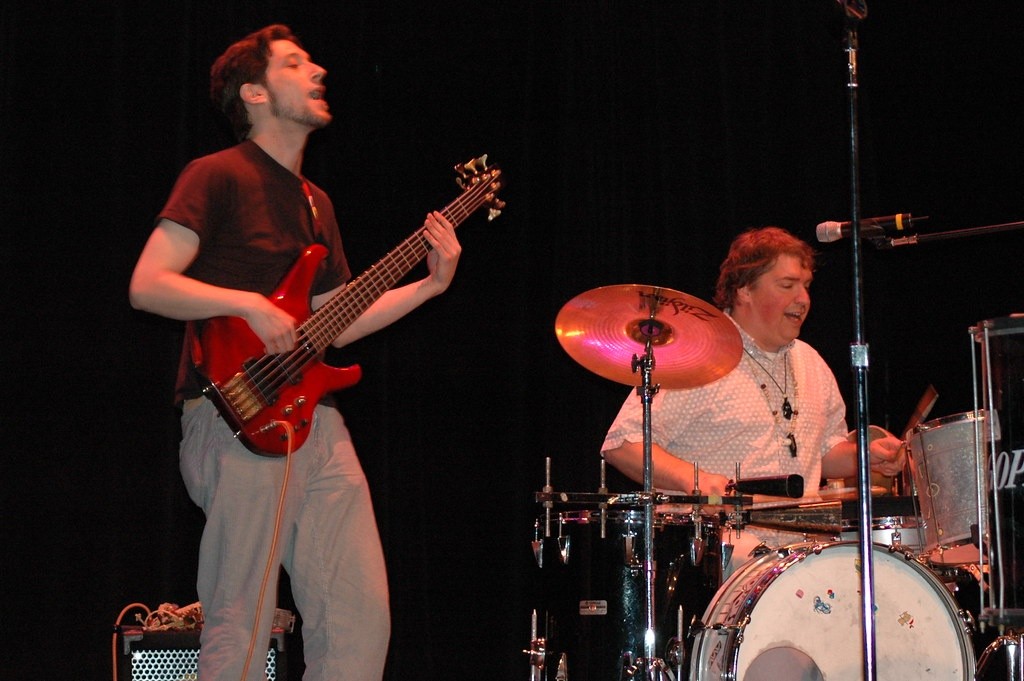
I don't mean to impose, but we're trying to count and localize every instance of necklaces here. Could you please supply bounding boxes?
[743,347,799,457]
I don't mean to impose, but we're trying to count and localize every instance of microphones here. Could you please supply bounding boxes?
[815,213,929,244]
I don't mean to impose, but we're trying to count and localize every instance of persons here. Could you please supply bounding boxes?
[129,24,461,681]
[600,226,1008,681]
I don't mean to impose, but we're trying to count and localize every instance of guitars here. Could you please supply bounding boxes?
[185,154,508,458]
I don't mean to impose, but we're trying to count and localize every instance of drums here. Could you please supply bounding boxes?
[687,539,980,681]
[968,313,1024,630]
[901,409,988,568]
[530,508,730,681]
[838,514,966,600]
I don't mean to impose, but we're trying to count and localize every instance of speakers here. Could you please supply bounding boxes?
[118,625,288,681]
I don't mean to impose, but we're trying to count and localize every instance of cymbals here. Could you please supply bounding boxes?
[553,282,742,393]
